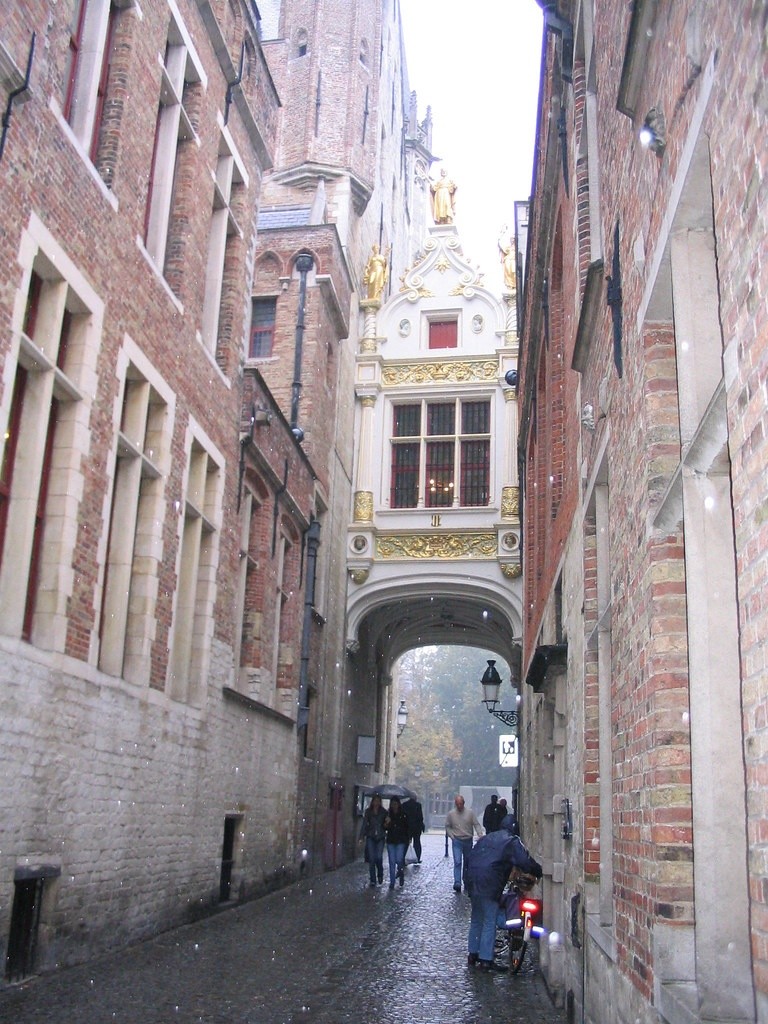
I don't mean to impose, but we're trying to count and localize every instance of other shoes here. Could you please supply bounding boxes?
[400,878,404,885]
[378,877,383,884]
[389,884,394,889]
[370,881,376,886]
[468,954,481,964]
[453,884,461,892]
[482,961,508,973]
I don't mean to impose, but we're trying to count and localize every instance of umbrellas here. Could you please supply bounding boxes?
[364,784,416,817]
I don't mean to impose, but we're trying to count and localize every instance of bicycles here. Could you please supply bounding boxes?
[494,870,546,976]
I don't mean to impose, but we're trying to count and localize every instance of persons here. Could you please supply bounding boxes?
[358,795,388,888]
[429,169,456,225]
[483,795,508,835]
[445,795,483,892]
[364,243,389,299]
[385,797,412,889]
[503,235,516,289]
[463,814,542,973]
[401,791,425,866]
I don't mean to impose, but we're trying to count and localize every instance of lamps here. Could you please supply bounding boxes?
[478,660,521,726]
[397,700,408,738]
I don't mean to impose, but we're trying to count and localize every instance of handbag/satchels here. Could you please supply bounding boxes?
[405,840,418,865]
